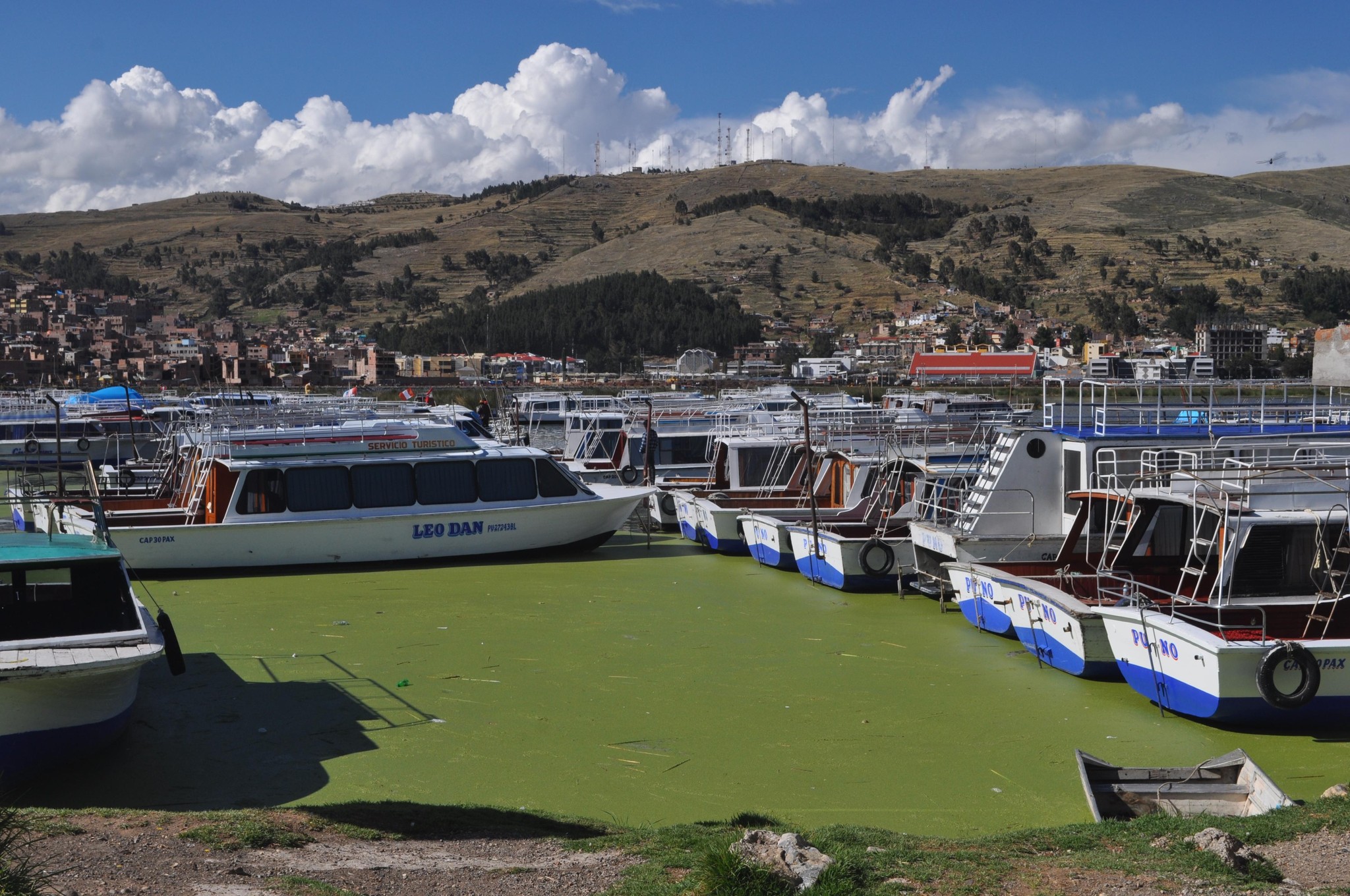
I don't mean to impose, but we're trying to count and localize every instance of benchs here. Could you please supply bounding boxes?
[1094,782,1253,793]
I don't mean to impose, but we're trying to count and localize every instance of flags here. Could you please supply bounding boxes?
[343,387,357,397]
[399,388,414,401]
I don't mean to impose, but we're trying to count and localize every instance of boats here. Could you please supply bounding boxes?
[1,499,168,742]
[0,387,659,571]
[1072,747,1301,824]
[498,347,1350,739]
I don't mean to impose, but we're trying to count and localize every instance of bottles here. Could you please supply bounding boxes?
[1123,582,1129,596]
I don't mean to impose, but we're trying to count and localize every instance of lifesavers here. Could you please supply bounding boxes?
[857,539,895,575]
[77,438,90,451]
[115,469,136,488]
[785,531,794,552]
[621,465,638,483]
[737,521,746,541]
[156,613,187,676]
[1253,643,1322,708]
[660,494,677,516]
[24,440,41,455]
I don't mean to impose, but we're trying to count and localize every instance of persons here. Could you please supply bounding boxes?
[305,380,316,403]
[425,395,429,407]
[639,420,658,485]
[584,379,586,384]
[476,398,490,427]
[513,380,520,386]
